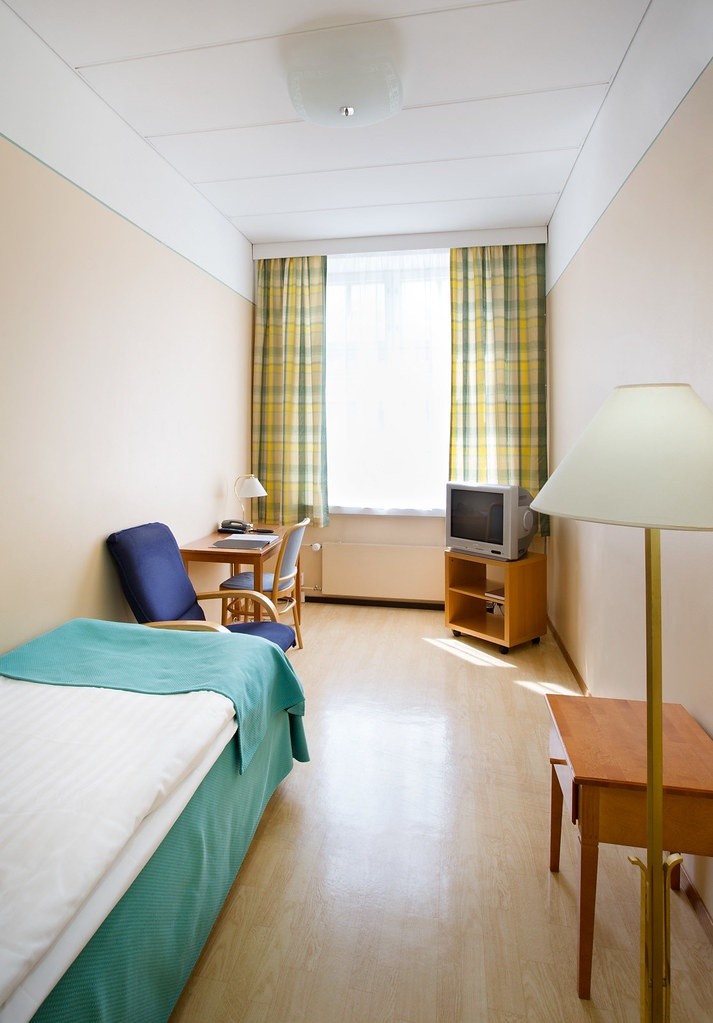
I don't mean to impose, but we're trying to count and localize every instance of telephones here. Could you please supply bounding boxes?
[221,519,253,530]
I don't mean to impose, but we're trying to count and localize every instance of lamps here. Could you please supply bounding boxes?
[525,380,713,1019]
[233,473,270,532]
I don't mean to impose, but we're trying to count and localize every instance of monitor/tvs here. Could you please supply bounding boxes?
[445,481,539,563]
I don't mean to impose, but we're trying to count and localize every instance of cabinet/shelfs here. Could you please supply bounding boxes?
[444,549,548,655]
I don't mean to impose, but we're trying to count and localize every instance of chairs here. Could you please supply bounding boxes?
[105,521,298,706]
[216,518,303,649]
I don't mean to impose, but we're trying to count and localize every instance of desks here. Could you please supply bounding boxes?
[174,520,303,630]
[543,690,712,1001]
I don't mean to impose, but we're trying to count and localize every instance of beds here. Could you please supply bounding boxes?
[1,616,312,1022]
